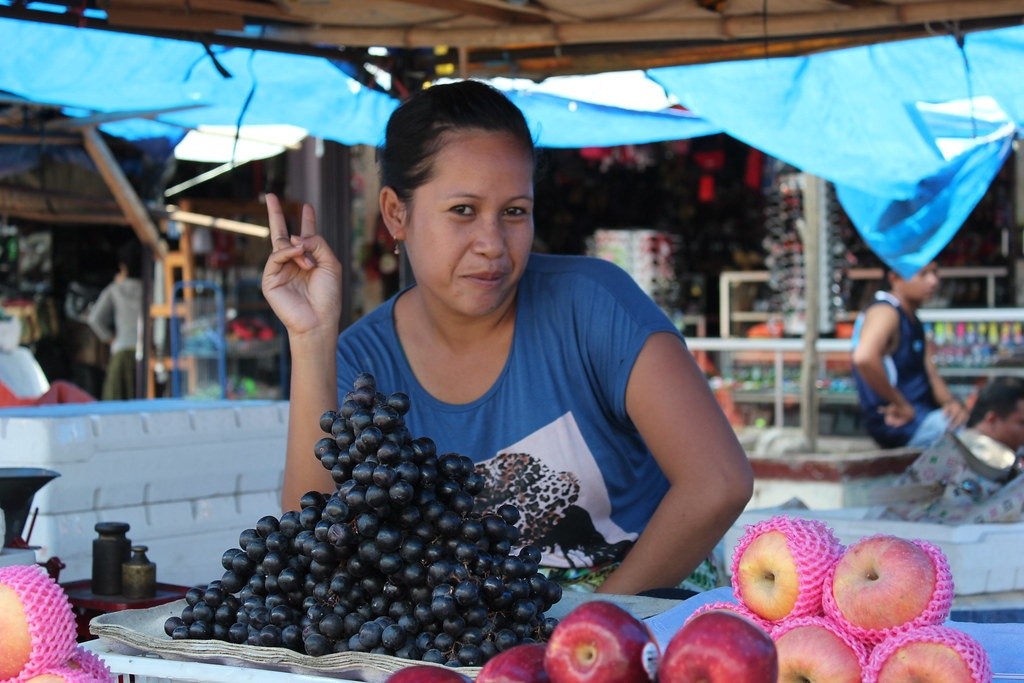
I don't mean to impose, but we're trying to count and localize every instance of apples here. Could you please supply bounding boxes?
[0,565,116,683]
[383,516,994,683]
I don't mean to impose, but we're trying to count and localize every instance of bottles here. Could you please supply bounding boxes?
[924,322,1023,369]
[122,546,157,598]
[92,522,132,595]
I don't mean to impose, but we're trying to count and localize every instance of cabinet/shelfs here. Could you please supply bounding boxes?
[680,268,1024,451]
[143,201,304,400]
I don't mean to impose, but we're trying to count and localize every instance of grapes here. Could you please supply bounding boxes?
[164,372,564,670]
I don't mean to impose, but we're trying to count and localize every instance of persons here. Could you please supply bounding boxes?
[88,257,142,401]
[965,378,1023,455]
[852,255,969,448]
[261,80,752,595]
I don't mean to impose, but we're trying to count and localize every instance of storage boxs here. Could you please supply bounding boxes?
[77,587,737,683]
[724,504,1024,595]
[0,397,287,586]
